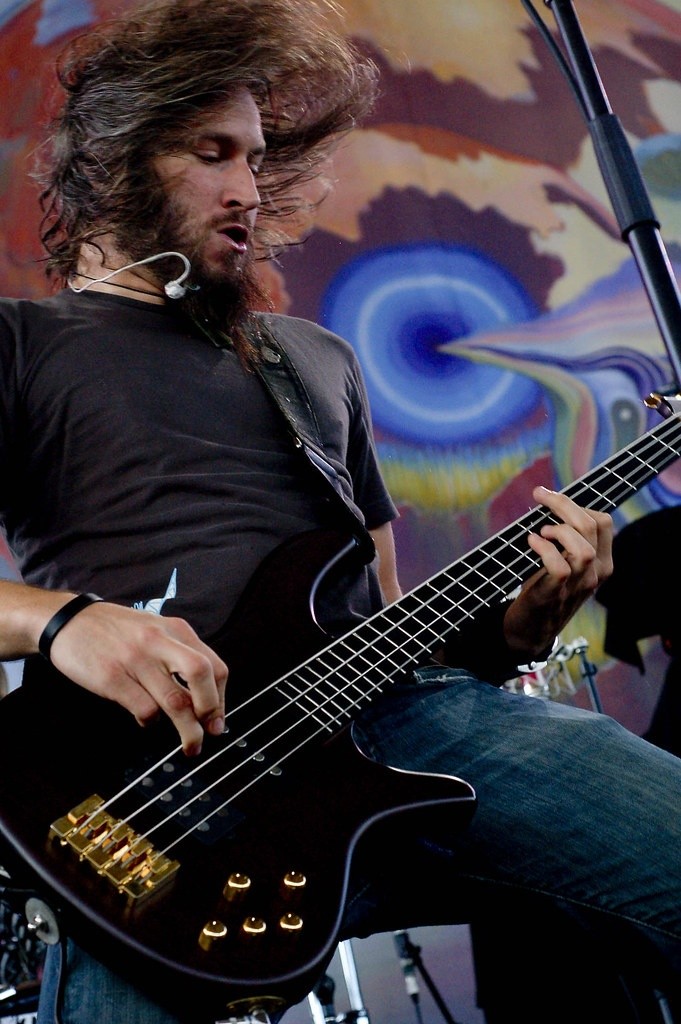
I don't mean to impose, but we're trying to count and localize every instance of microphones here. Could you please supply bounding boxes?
[392,932,420,1002]
[313,975,336,1024]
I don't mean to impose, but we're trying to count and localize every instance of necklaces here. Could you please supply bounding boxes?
[75,272,163,297]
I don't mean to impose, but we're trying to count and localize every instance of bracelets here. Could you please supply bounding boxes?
[487,597,558,675]
[38,594,104,660]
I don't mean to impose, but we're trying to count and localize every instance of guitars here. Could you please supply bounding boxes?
[0,391,681,1024]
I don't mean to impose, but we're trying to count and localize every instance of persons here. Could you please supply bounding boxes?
[1,0,680,1023]
